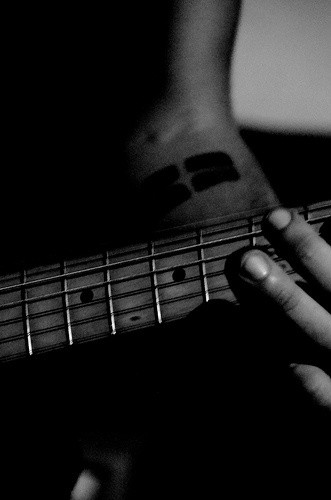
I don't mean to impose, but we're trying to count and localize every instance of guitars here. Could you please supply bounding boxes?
[0,195,330,358]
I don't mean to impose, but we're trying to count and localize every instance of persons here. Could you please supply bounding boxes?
[65,1,331,500]
